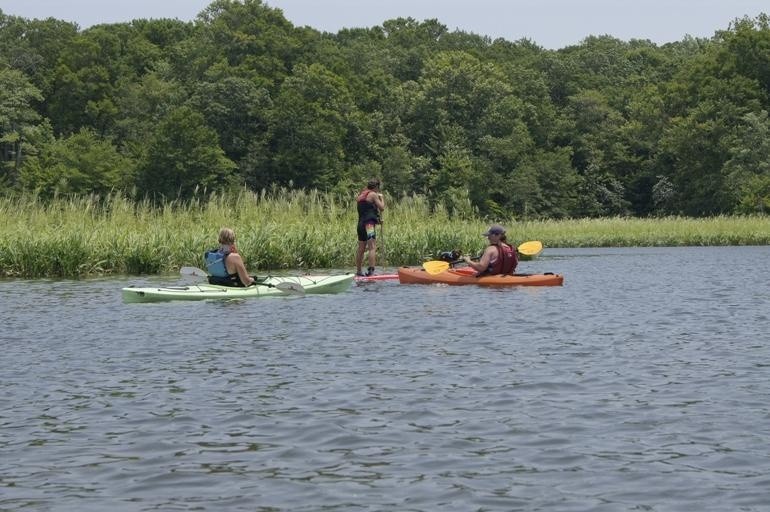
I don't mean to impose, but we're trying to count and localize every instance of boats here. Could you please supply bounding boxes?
[396,265,564,288]
[348,268,400,285]
[121,273,356,306]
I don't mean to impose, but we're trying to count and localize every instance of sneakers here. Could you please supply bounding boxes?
[357,270,376,278]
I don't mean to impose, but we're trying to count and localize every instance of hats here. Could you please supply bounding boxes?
[481,225,506,238]
[367,178,380,189]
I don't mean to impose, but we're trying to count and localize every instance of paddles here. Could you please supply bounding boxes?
[422,241,543,276]
[180,267,306,296]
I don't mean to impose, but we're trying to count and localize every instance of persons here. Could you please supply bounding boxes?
[462,224,519,276]
[203,227,257,288]
[355,178,384,277]
